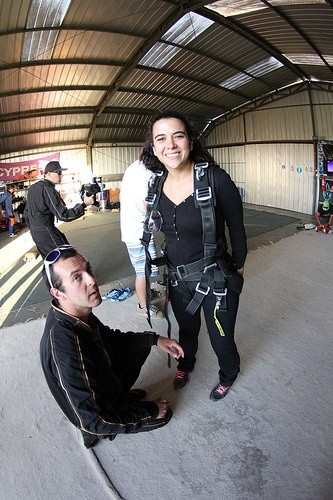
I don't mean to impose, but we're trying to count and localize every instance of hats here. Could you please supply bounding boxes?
[44,161,68,174]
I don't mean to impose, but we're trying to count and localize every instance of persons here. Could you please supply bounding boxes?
[0,184,19,238]
[119,158,165,320]
[24,160,94,260]
[39,244,184,449]
[140,111,248,401]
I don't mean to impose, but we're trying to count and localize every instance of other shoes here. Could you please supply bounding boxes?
[81,430,98,448]
[127,389,147,402]
[9,233,18,237]
[100,287,134,302]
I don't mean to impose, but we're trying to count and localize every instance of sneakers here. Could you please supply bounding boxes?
[210,383,230,402]
[137,303,164,319]
[173,369,192,391]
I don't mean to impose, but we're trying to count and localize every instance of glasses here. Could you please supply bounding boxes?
[44,244,75,288]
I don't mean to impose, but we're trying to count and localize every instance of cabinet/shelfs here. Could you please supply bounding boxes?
[6,179,33,225]
